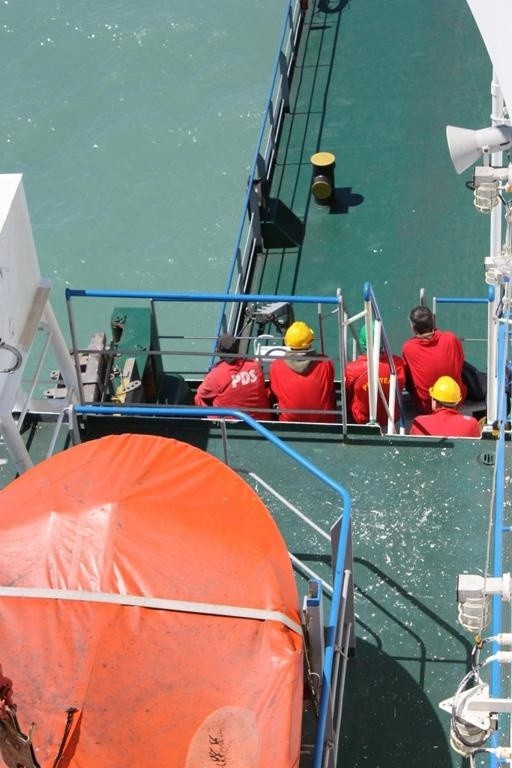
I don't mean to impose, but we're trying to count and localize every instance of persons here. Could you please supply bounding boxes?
[269,320,336,424]
[344,324,405,424]
[194,334,272,420]
[401,306,467,414]
[408,375,482,437]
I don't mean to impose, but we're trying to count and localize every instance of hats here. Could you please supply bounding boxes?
[217,334,237,352]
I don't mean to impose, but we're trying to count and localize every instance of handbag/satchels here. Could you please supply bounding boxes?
[462,361,487,400]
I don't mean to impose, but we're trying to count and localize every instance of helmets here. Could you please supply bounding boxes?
[359,321,383,349]
[429,375,462,403]
[284,321,314,349]
